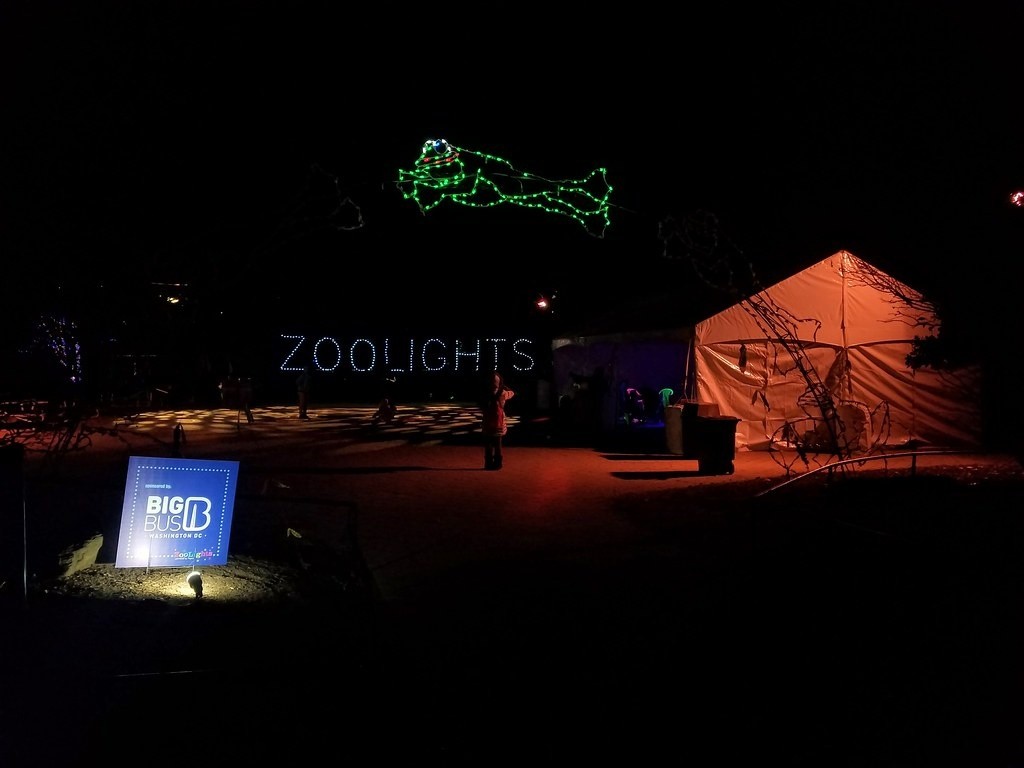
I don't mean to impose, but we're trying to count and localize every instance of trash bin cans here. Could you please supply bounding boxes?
[698,415,741,474]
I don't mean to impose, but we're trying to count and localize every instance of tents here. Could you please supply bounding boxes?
[534,236,996,456]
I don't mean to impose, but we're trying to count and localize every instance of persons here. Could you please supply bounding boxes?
[476,372,517,472]
